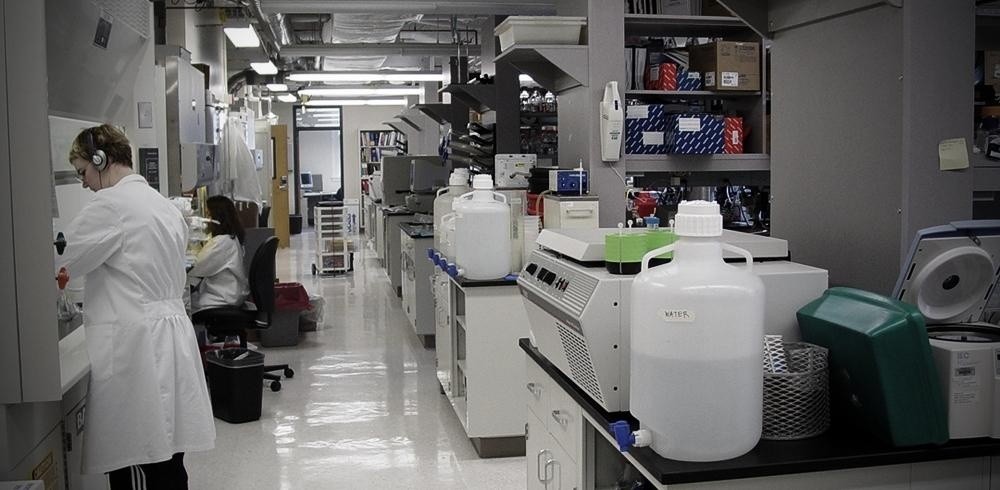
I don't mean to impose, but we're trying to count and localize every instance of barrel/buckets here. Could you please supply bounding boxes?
[433,211,454,265]
[426,170,468,262]
[440,216,455,270]
[615,199,766,464]
[448,176,511,281]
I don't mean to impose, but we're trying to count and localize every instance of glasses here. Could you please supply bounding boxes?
[72,160,93,184]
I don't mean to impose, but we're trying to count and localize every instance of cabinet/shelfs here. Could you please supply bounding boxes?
[398,221,434,347]
[434,265,533,458]
[624,14,772,155]
[167,54,206,140]
[519,337,583,490]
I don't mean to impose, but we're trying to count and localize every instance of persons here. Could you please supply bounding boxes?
[175,194,252,318]
[44,123,219,489]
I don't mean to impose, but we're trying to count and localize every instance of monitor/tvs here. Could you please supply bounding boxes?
[299,171,314,192]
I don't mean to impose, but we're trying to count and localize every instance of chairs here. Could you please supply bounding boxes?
[192,236,294,393]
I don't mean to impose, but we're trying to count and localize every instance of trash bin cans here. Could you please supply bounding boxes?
[261,282,301,346]
[203,347,266,424]
[289,214,302,234]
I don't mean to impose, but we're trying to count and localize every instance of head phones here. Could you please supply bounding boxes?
[86,128,108,171]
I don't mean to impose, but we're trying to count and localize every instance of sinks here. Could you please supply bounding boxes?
[53,300,83,340]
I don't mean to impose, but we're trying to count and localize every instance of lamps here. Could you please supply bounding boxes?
[223,20,263,48]
[249,57,280,77]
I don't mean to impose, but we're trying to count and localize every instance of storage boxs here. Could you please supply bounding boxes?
[696,42,760,92]
[625,103,743,154]
